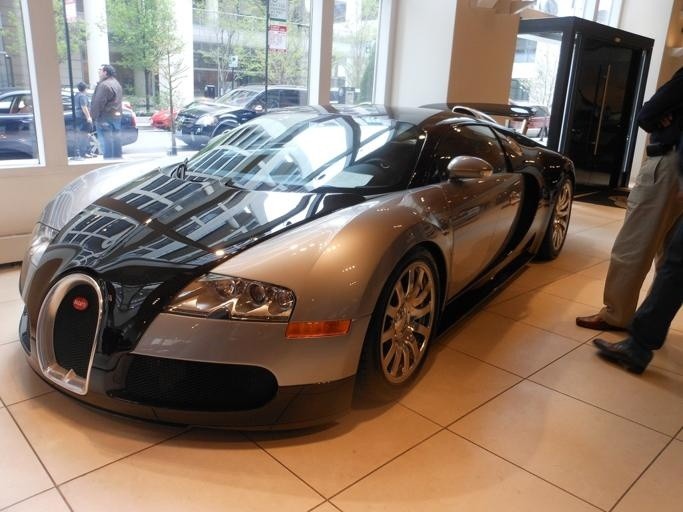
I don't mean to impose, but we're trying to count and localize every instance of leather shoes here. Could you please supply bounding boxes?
[576,312,627,331]
[592,337,654,375]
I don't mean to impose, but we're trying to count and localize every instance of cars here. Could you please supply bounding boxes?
[0,86,181,160]
[509,100,551,139]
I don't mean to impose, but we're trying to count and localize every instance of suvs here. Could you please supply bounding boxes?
[172,83,308,150]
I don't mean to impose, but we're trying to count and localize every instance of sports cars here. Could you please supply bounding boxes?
[8,97,579,437]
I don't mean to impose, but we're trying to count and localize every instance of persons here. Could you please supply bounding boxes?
[576,66,683,375]
[74,64,124,158]
